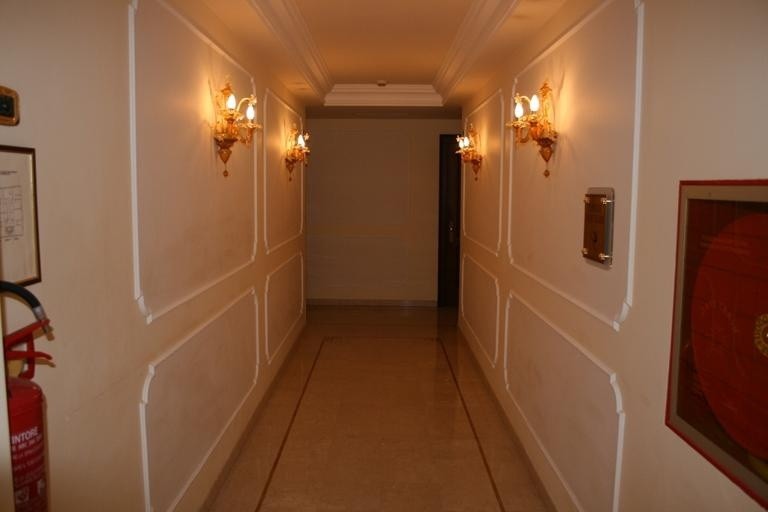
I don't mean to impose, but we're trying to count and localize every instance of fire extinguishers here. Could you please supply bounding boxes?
[0,280,56,512]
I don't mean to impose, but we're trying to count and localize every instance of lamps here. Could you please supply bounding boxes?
[201,82,312,180]
[455,86,562,183]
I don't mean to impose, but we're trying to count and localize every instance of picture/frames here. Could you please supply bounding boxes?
[662,177,768,512]
[0,145,41,290]
